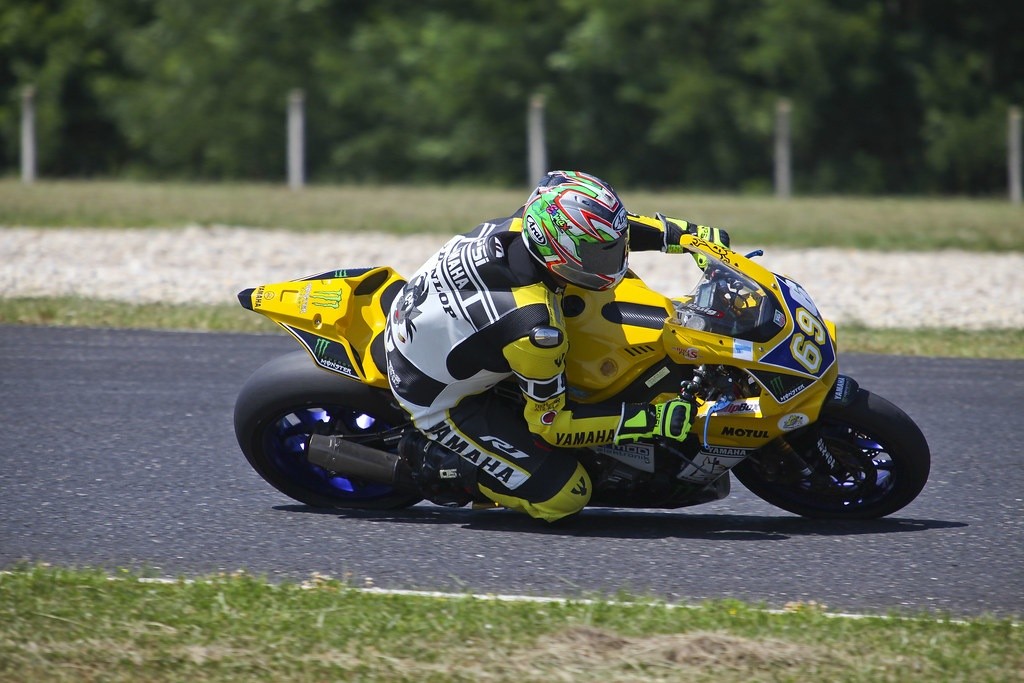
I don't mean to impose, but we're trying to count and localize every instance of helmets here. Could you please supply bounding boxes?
[521,170,630,291]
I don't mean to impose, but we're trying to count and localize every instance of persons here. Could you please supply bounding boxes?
[383,168,730,524]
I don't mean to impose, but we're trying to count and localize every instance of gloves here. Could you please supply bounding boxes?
[655,212,730,255]
[612,397,698,446]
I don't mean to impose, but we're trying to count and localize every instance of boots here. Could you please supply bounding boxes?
[399,431,479,509]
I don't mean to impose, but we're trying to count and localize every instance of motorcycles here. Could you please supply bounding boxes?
[232,236,932,528]
